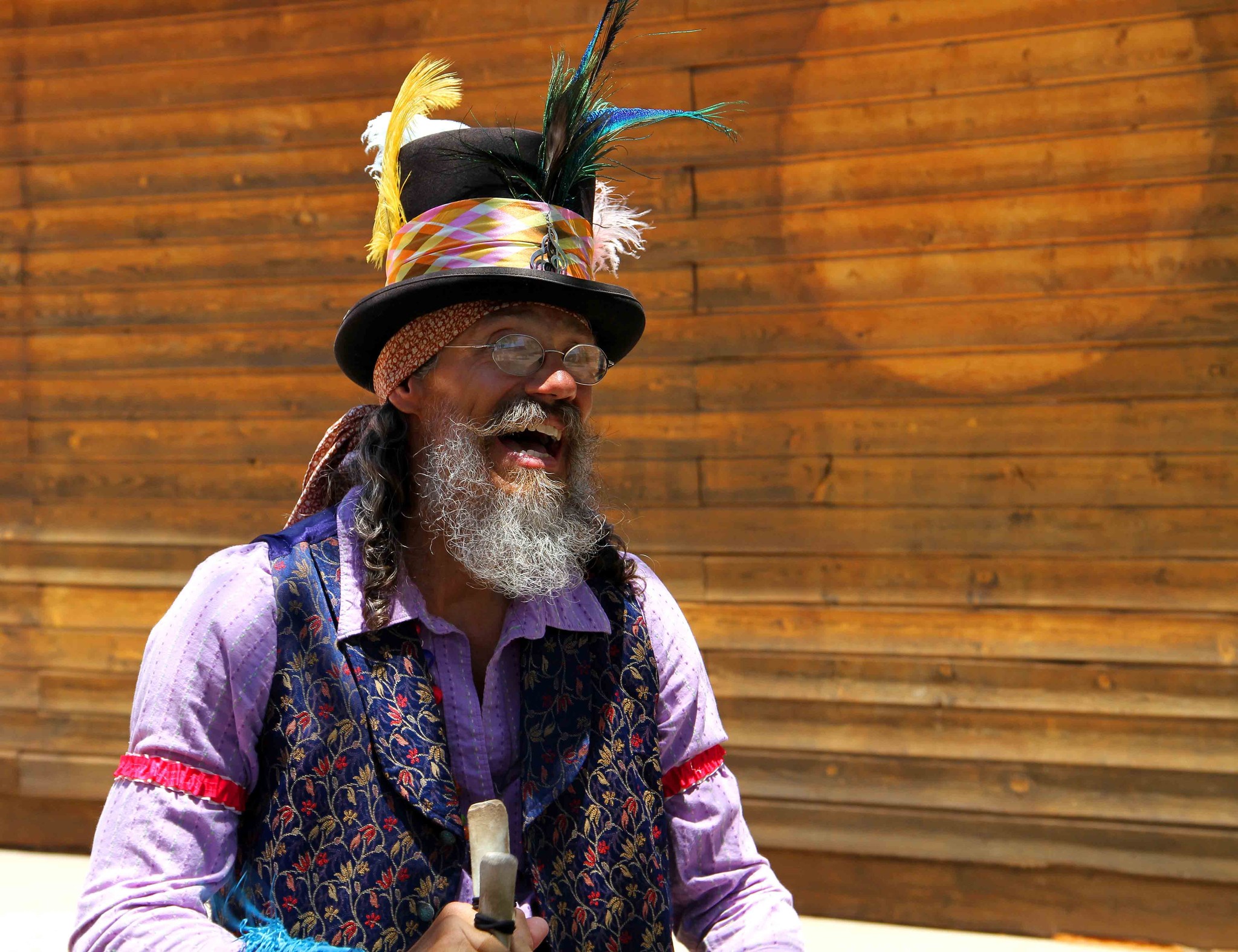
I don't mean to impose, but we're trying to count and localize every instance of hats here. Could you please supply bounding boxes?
[332,0,748,393]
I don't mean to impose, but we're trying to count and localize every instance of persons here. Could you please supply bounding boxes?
[68,127,804,952]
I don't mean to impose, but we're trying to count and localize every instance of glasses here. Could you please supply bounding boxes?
[444,333,614,387]
